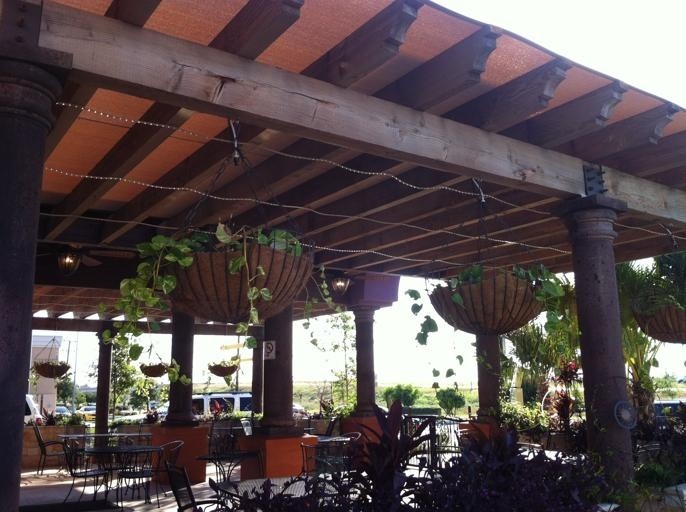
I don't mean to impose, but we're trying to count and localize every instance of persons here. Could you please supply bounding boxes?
[211,399,221,414]
[191,403,202,415]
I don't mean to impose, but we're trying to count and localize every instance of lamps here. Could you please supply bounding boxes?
[331,270,351,297]
[51,244,84,278]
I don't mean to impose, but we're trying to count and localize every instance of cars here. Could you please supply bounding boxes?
[24,394,96,426]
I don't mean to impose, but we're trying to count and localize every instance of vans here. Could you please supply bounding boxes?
[156,392,253,425]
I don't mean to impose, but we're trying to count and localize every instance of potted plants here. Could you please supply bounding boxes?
[96,220,318,397]
[626,295,686,418]
[399,256,573,415]
[26,359,73,402]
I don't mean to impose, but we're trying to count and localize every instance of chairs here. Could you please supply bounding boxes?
[55,413,184,512]
[200,414,363,511]
[166,463,230,512]
[32,419,72,475]
